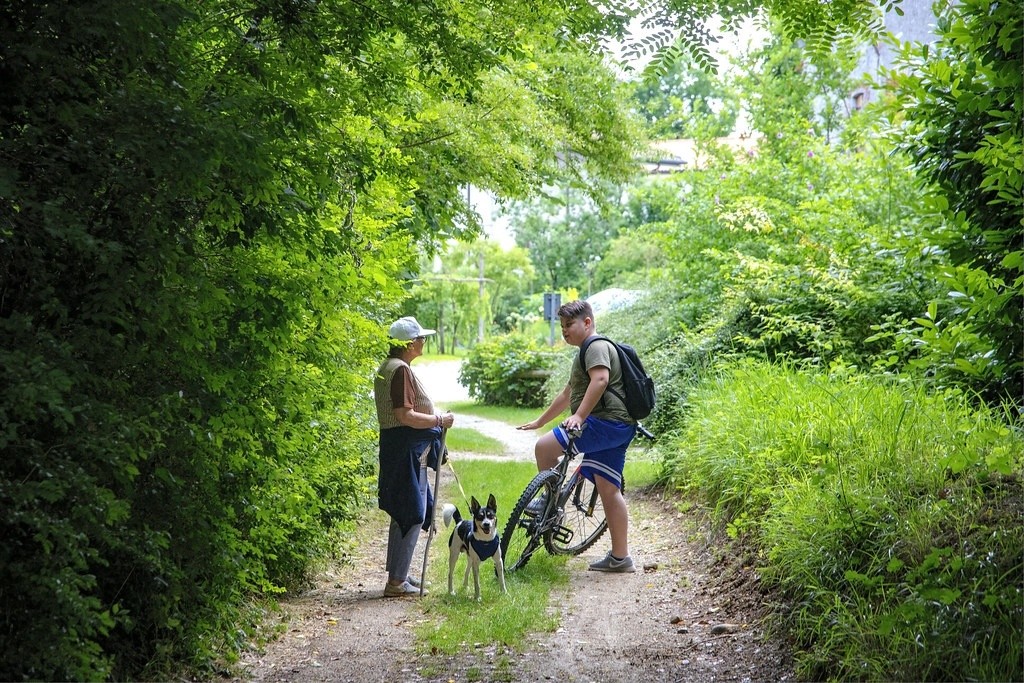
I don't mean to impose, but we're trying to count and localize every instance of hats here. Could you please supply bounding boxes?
[388,317,437,341]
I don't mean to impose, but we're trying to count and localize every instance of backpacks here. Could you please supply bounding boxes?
[580,335,656,420]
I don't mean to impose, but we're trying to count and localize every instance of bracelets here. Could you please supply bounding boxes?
[434,415,439,428]
[440,415,443,426]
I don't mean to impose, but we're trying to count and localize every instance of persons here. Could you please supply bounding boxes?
[515,300,637,574]
[374,316,454,596]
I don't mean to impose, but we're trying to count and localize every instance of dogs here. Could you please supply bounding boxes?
[443,494,506,602]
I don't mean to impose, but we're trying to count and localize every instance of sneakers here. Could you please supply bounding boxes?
[406,575,431,587]
[589,550,636,572]
[384,581,429,596]
[524,492,558,514]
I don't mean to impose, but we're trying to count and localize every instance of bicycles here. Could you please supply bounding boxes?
[494,421,654,579]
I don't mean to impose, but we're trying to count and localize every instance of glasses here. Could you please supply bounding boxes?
[416,337,427,344]
